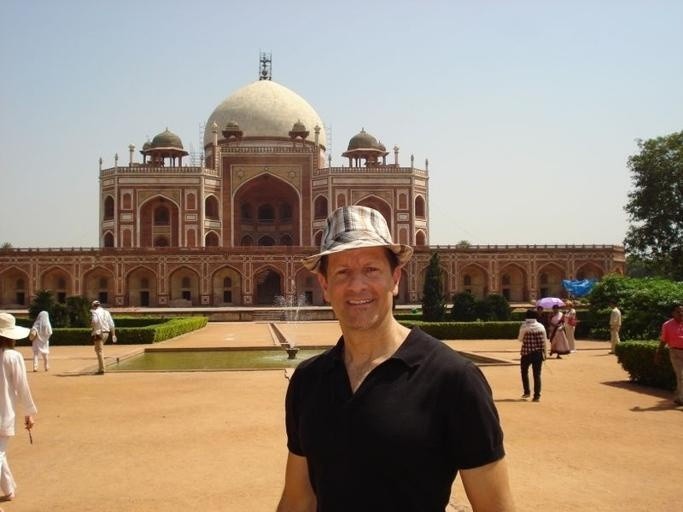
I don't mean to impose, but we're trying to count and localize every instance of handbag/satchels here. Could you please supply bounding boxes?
[29,327,38,341]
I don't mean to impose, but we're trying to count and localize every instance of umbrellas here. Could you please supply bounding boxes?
[536,298,566,312]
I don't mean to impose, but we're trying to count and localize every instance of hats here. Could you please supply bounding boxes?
[299,205,413,276]
[92,300,100,306]
[0,312,30,341]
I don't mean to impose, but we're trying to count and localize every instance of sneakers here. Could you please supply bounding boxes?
[2,493,14,501]
[93,371,104,376]
[520,394,542,403]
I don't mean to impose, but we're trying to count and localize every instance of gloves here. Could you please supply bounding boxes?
[111,335,117,343]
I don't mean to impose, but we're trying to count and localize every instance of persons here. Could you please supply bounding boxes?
[608,299,622,354]
[518,310,547,402]
[31,311,52,372]
[655,303,683,410]
[548,305,570,359]
[92,300,117,374]
[275,204,518,512]
[0,313,38,501]
[537,306,548,330]
[564,300,576,352]
